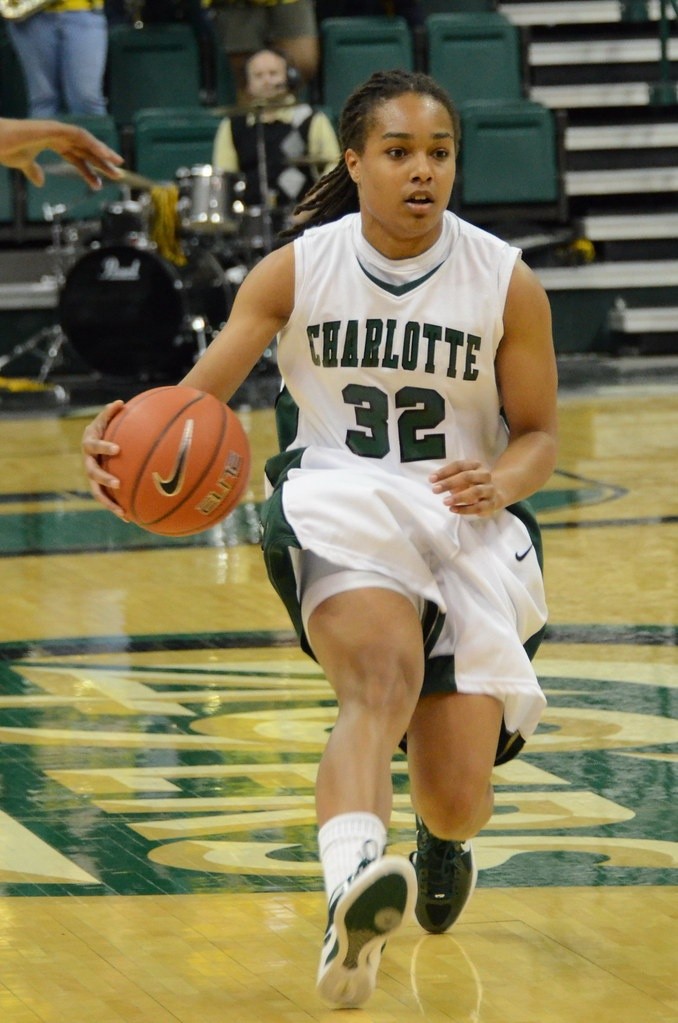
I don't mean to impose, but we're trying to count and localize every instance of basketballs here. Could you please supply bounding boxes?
[98,384,251,539]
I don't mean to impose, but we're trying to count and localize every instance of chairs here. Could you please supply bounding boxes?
[0,0,570,419]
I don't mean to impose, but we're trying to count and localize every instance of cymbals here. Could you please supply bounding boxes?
[208,95,302,116]
[279,154,342,166]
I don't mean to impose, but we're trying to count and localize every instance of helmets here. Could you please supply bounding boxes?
[103,200,143,237]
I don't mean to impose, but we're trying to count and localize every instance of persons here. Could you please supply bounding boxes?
[83,73,559,1009]
[1,1,346,240]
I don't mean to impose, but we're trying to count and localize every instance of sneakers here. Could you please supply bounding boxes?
[314,839,419,1009]
[409,815,477,935]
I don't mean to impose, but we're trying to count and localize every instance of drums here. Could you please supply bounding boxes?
[175,163,246,235]
[233,205,290,249]
[58,237,235,383]
[101,199,156,252]
